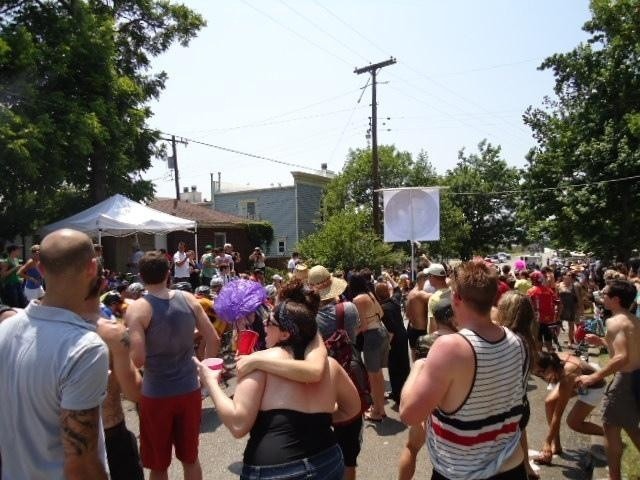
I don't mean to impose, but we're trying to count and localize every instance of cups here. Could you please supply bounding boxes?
[238,330,259,355]
[201,357,223,387]
[588,334,596,347]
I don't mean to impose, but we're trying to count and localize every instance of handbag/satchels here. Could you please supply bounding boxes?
[571,289,578,303]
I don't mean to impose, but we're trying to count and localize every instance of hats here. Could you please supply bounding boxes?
[423,262,447,277]
[6,244,25,253]
[528,271,544,282]
[272,274,282,279]
[205,245,212,249]
[400,274,407,280]
[377,276,385,282]
[94,244,104,249]
[301,265,348,300]
[429,287,452,314]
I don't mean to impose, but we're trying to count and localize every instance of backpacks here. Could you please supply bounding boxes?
[324,302,372,413]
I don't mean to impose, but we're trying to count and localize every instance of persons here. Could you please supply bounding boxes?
[1,228,640,480]
[388,190,427,239]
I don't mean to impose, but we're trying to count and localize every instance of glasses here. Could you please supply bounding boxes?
[266,315,281,328]
[603,290,615,296]
[563,274,572,276]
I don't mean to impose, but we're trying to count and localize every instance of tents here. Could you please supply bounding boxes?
[36,193,198,263]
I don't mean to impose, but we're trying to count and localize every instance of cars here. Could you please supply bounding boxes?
[522,255,574,272]
[556,248,585,259]
[485,251,511,272]
